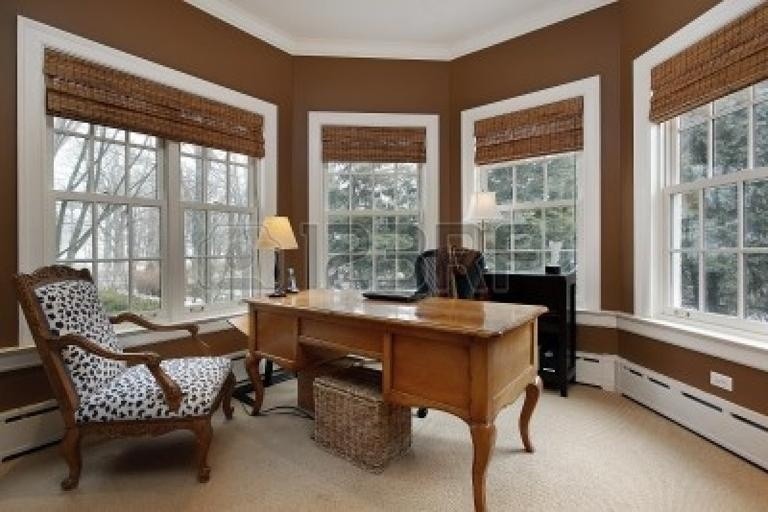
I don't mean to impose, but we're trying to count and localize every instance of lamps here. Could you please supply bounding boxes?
[254,216,298,298]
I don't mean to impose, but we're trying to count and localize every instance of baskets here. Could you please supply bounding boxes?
[312,365,414,477]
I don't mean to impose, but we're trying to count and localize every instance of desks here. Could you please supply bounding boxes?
[241,288,549,512]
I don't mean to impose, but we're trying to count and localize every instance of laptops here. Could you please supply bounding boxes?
[363,289,427,301]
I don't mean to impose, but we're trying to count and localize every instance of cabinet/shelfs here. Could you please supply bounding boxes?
[483,269,576,398]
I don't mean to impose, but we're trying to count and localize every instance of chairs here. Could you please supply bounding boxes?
[12,262,237,492]
[415,249,489,301]
